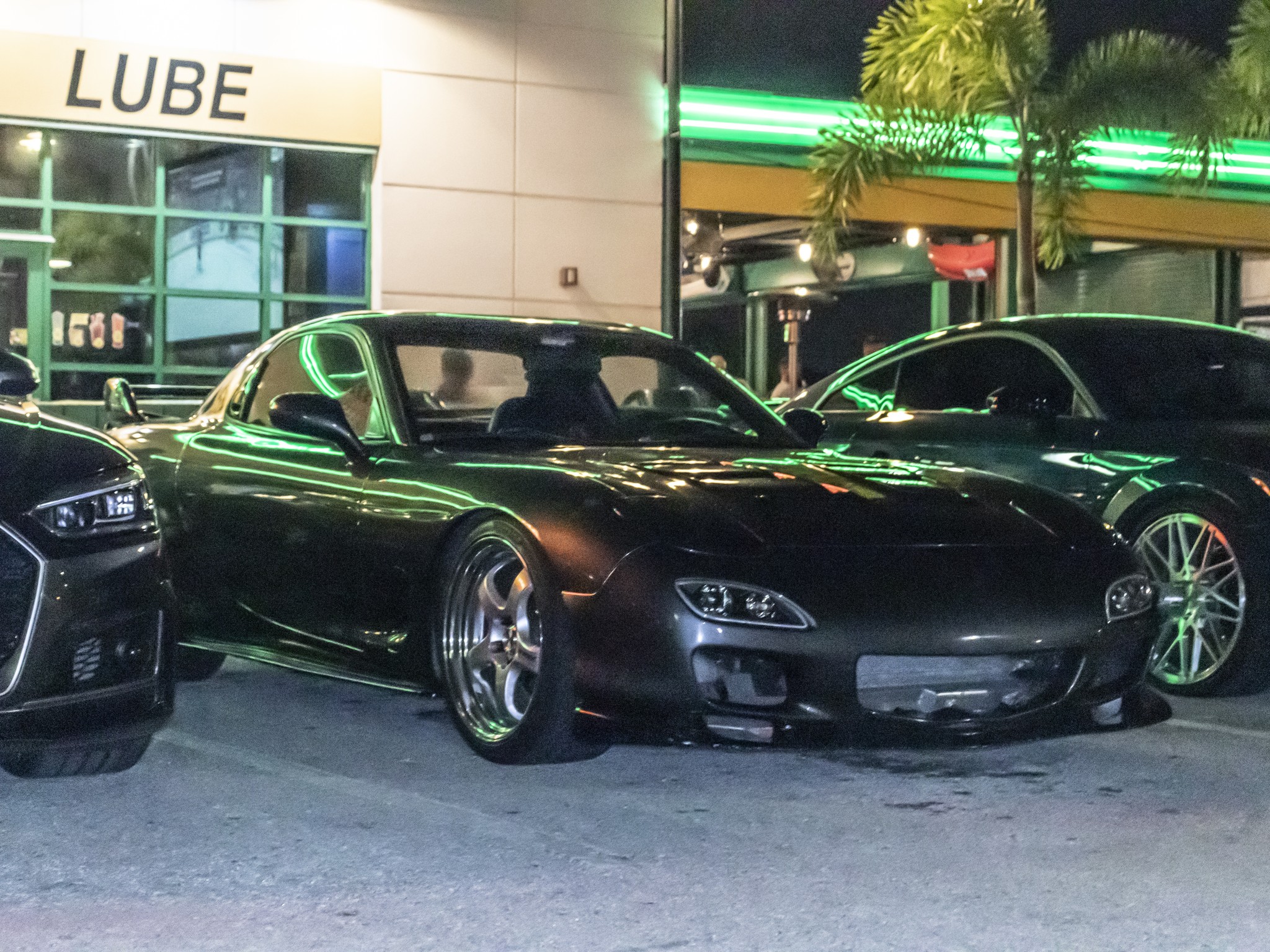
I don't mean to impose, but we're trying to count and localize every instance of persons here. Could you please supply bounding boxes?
[769,354,806,404]
[710,354,727,371]
[432,346,473,407]
[861,334,913,405]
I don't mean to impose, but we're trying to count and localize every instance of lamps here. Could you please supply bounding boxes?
[560,264,579,289]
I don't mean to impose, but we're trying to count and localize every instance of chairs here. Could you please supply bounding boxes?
[483,394,552,442]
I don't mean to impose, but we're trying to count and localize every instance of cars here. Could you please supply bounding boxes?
[0,347,178,781]
[753,312,1269,697]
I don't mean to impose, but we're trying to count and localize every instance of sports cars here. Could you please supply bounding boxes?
[108,309,1162,767]
